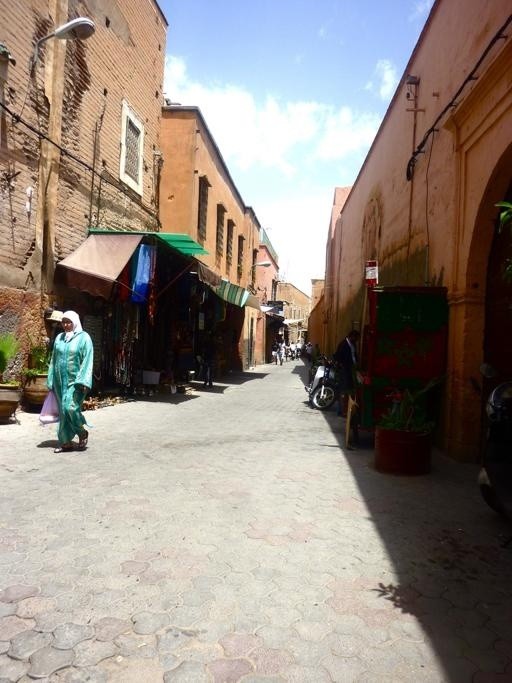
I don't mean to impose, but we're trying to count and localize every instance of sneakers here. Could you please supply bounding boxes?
[79,430,88,448]
[54,442,74,453]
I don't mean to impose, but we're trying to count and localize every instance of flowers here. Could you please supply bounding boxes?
[375,386,431,432]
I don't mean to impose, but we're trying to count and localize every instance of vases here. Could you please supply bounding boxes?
[374,429,434,474]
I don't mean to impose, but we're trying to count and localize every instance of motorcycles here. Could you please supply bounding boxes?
[469,363,512,522]
[291,351,302,360]
[305,353,339,409]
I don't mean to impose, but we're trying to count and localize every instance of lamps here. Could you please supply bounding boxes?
[29,15,97,67]
[249,260,272,275]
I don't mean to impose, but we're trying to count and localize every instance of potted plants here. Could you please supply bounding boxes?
[0,330,54,423]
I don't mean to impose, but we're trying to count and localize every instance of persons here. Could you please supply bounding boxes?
[46,310,94,453]
[272,340,312,365]
[333,330,359,417]
